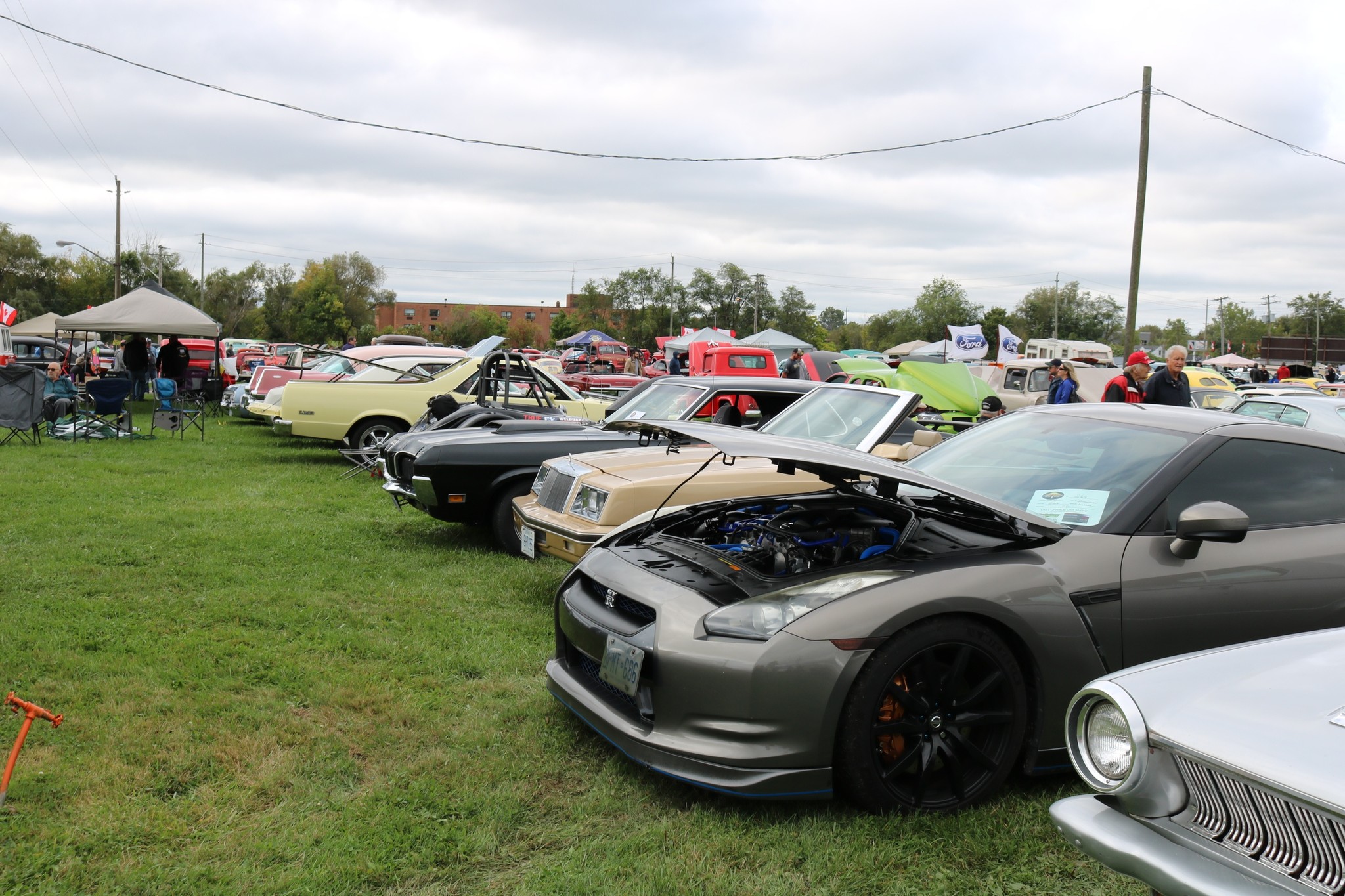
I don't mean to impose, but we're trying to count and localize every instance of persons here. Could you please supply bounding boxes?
[44,333,235,423]
[493,344,1335,424]
[327,336,378,356]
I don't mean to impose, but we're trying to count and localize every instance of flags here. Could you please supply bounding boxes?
[713,326,736,338]
[997,324,1024,361]
[681,326,698,337]
[1211,341,1260,352]
[946,324,989,359]
[87,305,94,309]
[0,301,17,325]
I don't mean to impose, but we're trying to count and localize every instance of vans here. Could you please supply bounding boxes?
[0,324,17,368]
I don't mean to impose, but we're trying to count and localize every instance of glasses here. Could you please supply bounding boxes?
[46,368,60,371]
[1059,367,1066,370]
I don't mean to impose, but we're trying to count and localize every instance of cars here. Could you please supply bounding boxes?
[11,336,227,381]
[1048,627,1345,896]
[544,402,1345,818]
[210,334,1105,564]
[973,356,1345,438]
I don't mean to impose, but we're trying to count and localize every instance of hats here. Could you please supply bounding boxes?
[75,357,86,364]
[981,395,1007,411]
[1126,351,1157,365]
[1045,358,1062,367]
[584,351,590,356]
[793,348,802,356]
[146,337,152,343]
[120,340,126,344]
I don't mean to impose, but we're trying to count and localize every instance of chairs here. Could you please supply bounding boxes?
[0,362,223,444]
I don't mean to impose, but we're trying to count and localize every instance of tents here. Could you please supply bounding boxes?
[665,327,813,363]
[9,278,223,403]
[883,339,953,356]
[555,329,618,361]
[1201,353,1261,371]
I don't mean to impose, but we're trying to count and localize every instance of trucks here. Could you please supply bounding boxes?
[1024,338,1112,374]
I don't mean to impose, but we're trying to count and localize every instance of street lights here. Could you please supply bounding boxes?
[736,297,756,334]
[56,240,122,351]
[140,266,161,347]
